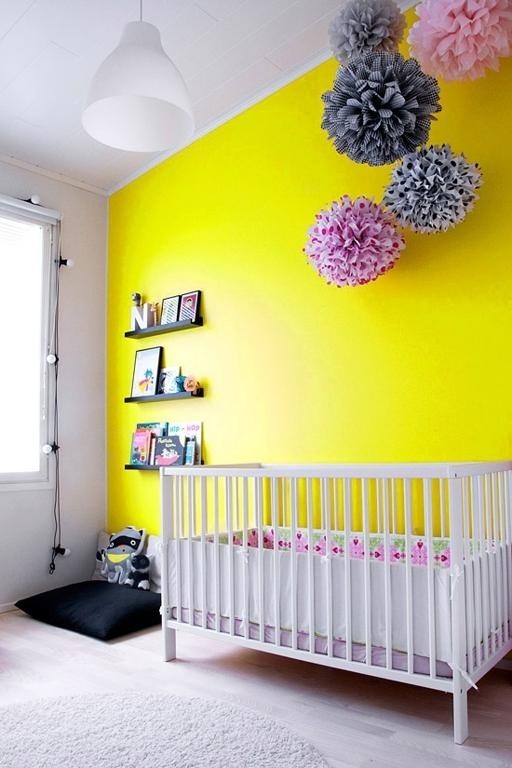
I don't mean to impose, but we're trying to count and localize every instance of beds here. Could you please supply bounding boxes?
[161,460,512,746]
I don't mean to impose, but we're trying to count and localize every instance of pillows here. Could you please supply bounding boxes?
[14,579,161,643]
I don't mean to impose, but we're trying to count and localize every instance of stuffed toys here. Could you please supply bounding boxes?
[95,527,151,589]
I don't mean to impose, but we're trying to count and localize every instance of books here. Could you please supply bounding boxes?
[129,422,202,466]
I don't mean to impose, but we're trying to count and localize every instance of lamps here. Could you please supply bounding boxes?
[77,0,198,153]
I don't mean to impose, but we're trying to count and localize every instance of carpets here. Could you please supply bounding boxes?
[1,691,333,768]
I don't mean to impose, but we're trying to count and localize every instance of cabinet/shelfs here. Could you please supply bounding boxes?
[124,318,203,471]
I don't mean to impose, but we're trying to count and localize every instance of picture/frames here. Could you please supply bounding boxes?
[177,290,201,322]
[130,346,163,397]
[159,295,180,325]
